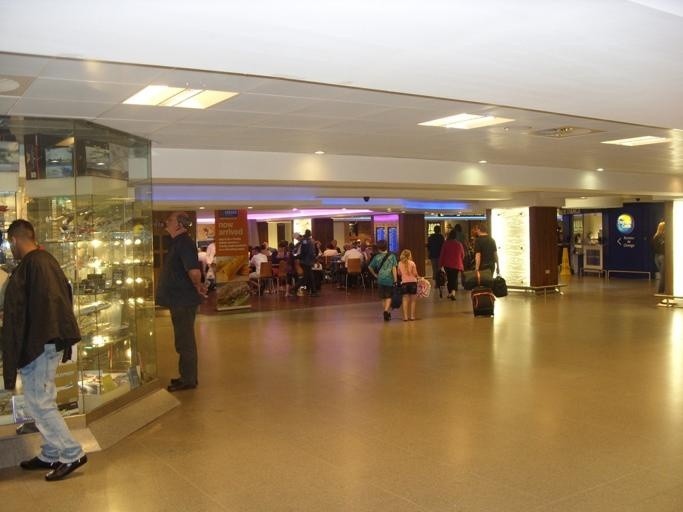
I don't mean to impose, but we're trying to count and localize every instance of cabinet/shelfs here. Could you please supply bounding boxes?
[0,113,162,438]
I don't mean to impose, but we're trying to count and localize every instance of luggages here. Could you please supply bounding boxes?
[471,285,495,318]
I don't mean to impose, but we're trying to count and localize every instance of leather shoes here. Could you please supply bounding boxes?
[20,450,63,472]
[45,453,88,482]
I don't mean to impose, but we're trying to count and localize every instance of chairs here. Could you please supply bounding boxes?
[247,244,375,305]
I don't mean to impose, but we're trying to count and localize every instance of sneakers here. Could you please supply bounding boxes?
[167,379,198,393]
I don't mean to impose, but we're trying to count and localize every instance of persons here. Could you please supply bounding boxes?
[557,227,602,265]
[196,229,324,305]
[314,236,423,321]
[155,212,208,392]
[427,223,499,300]
[653,221,665,297]
[0,219,88,481]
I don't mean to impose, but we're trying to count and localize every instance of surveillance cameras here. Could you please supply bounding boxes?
[636,198,640,201]
[364,197,369,201]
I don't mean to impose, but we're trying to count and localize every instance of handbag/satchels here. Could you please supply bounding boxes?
[492,276,508,298]
[368,268,377,279]
[462,268,492,288]
[389,283,404,309]
[435,269,446,286]
[416,278,431,297]
